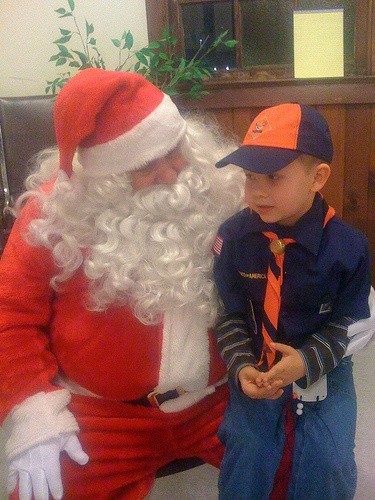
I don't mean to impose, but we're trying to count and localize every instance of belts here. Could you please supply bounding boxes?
[118,387,191,409]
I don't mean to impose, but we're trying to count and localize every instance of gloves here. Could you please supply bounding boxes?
[5,430,89,500]
[343,286,375,359]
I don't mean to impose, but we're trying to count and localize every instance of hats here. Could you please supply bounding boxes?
[215,104,334,173]
[53,67,186,190]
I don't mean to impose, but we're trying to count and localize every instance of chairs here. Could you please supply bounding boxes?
[1,94,209,478]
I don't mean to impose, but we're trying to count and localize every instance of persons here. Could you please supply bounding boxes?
[0,68,297,499]
[207,101,372,500]
